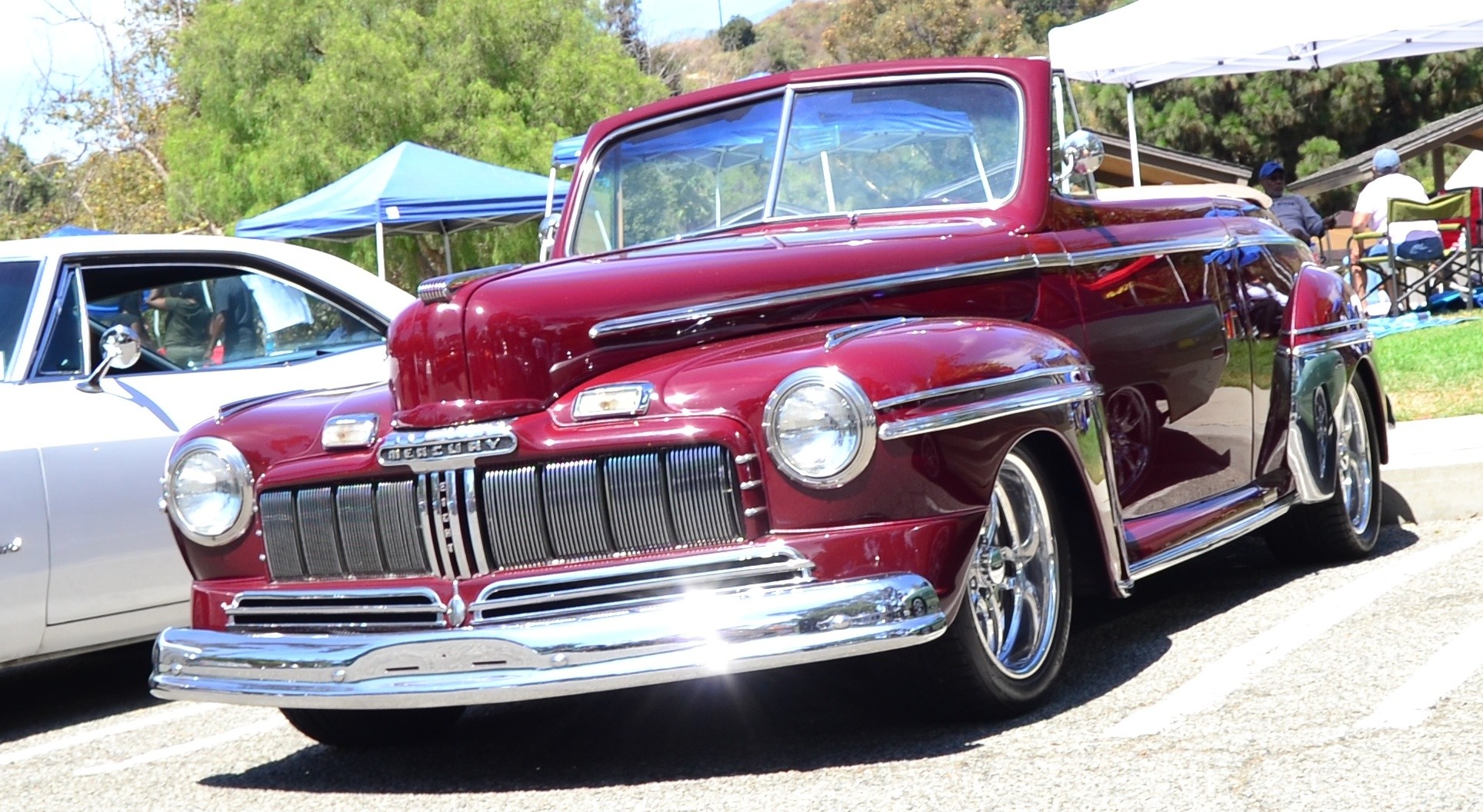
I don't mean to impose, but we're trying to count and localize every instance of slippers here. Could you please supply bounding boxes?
[1387,308,1403,317]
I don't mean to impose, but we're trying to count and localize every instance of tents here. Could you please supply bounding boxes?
[1203,245,1294,299]
[537,72,993,260]
[1047,0,1483,198]
[44,224,214,342]
[237,140,571,283]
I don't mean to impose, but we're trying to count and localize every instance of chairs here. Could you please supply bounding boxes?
[1317,187,1483,315]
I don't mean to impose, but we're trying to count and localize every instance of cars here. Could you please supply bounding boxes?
[150,55,1397,763]
[0,227,420,674]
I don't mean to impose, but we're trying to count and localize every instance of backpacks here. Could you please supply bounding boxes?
[1419,286,1483,313]
[1248,297,1285,336]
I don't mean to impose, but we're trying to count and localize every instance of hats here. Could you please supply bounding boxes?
[1374,149,1400,173]
[1259,162,1285,180]
[179,283,199,298]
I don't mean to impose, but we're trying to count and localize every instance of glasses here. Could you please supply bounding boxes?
[1267,174,1284,181]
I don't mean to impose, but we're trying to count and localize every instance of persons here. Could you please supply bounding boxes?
[1349,147,1444,318]
[147,281,215,371]
[204,276,258,364]
[1260,163,1337,248]
[86,293,151,343]
